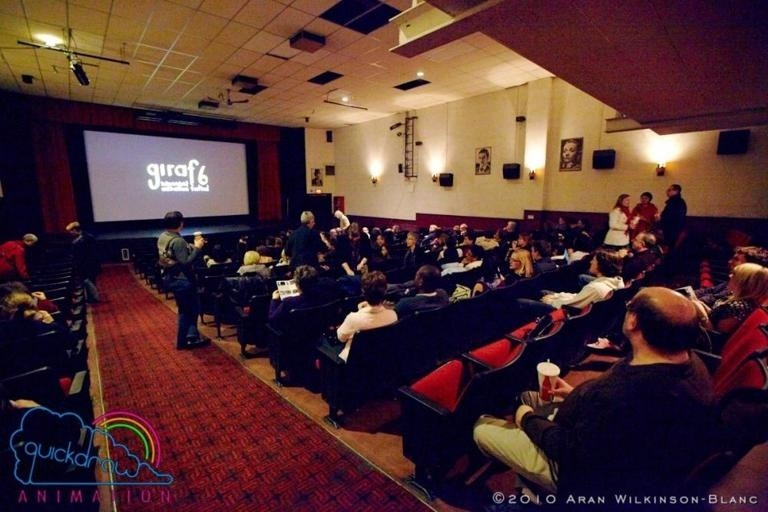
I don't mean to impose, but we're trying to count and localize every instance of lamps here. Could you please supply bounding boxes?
[17,28,129,85]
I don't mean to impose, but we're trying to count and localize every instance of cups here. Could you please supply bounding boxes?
[192,231,202,240]
[630,216,640,225]
[536,362,560,405]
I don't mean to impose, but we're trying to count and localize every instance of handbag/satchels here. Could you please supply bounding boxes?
[159,267,193,291]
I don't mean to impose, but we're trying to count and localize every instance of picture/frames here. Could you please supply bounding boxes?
[559,137,583,171]
[311,168,324,187]
[475,147,491,175]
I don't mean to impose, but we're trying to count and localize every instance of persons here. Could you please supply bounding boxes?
[1,183,768,512]
[313,168,320,184]
[473,149,491,174]
[556,137,584,172]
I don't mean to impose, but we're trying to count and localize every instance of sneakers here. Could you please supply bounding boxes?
[176,337,210,349]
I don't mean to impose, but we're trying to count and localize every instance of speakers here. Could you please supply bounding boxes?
[716,129,750,155]
[503,163,520,179]
[439,174,453,186]
[593,150,615,169]
